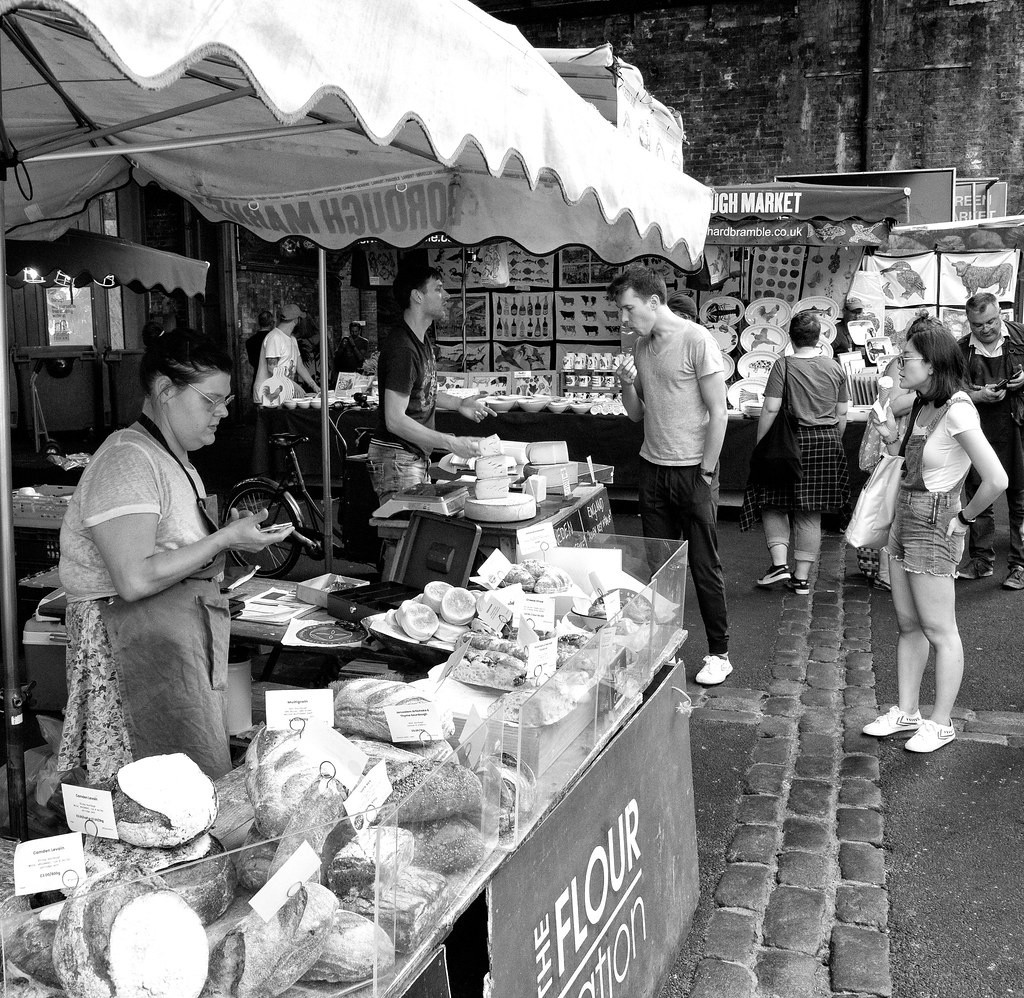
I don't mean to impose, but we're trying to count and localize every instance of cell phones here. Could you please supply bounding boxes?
[258,522,294,534]
[1008,370,1022,384]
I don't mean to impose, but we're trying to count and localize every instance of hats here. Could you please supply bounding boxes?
[844,297,865,311]
[280,304,307,321]
[668,294,697,318]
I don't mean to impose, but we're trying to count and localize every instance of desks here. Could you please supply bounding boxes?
[368,462,615,590]
[263,388,873,508]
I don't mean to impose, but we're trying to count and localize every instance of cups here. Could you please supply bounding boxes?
[565,392,622,402]
[563,353,623,370]
[566,376,622,388]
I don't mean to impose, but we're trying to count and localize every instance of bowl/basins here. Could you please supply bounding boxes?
[285,397,377,409]
[478,396,593,414]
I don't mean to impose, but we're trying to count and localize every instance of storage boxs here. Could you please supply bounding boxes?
[296,573,370,608]
[22,616,68,712]
[327,509,483,624]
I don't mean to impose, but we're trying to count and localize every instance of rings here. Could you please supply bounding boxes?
[996,396,999,399]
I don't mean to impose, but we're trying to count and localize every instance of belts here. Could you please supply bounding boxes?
[370,438,404,450]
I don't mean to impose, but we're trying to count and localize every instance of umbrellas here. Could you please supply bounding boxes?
[1,0,713,260]
[707,180,911,300]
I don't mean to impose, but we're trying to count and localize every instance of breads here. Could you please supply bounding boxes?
[0,561,654,998]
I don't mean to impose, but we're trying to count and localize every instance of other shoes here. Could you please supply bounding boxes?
[873,574,891,592]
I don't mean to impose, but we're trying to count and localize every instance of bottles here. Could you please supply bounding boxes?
[497,296,548,337]
[372,381,379,406]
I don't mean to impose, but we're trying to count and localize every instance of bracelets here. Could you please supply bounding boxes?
[701,469,714,477]
[883,434,899,445]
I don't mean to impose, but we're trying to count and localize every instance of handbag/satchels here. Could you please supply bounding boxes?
[750,356,803,484]
[844,397,924,551]
[858,356,900,475]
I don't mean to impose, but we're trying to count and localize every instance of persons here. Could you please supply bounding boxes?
[367,264,497,504]
[739,313,848,594]
[55,328,294,794]
[959,292,1024,592]
[332,322,368,386]
[860,327,1009,752]
[605,268,729,687]
[246,305,320,479]
[860,312,947,592]
[670,295,696,319]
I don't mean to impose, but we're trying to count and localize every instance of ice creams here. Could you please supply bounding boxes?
[878,376,893,408]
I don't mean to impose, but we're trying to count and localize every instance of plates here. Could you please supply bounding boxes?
[699,296,744,397]
[728,297,791,419]
[848,320,900,374]
[259,367,294,408]
[785,296,839,359]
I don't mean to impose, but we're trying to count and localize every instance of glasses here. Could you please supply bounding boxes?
[972,307,1001,332]
[845,308,861,315]
[897,355,923,367]
[156,371,235,413]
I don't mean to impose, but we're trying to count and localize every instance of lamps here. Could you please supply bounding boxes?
[22,267,46,284]
[54,269,75,288]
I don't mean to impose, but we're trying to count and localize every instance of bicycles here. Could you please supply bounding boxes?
[222,395,387,579]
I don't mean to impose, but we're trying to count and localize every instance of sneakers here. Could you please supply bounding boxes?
[863,706,922,736]
[784,571,809,595]
[1003,565,1024,589]
[696,652,733,684]
[956,558,994,579]
[756,563,791,588]
[905,718,955,752]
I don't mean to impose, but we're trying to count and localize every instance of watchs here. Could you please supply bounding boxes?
[958,511,976,525]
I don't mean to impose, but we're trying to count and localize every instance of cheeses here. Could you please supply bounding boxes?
[464,434,579,522]
